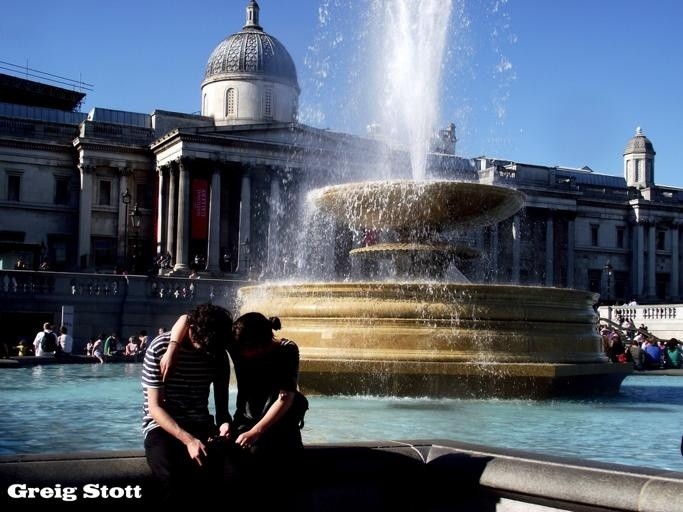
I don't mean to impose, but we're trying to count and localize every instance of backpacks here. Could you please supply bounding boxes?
[41,331,58,353]
[22,346,30,356]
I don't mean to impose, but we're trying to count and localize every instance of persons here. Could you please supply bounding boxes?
[160,305,303,512]
[12,321,170,366]
[141,301,235,512]
[152,252,171,269]
[600,318,683,370]
[15,259,26,282]
[189,270,201,291]
[38,261,50,271]
[191,255,206,272]
[222,245,237,272]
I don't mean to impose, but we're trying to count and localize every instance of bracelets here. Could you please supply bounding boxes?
[169,339,182,348]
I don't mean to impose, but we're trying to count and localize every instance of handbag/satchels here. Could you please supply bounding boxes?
[262,390,309,434]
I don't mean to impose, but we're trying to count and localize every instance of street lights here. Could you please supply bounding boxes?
[122,188,142,275]
[240,237,251,272]
[604,260,613,300]
